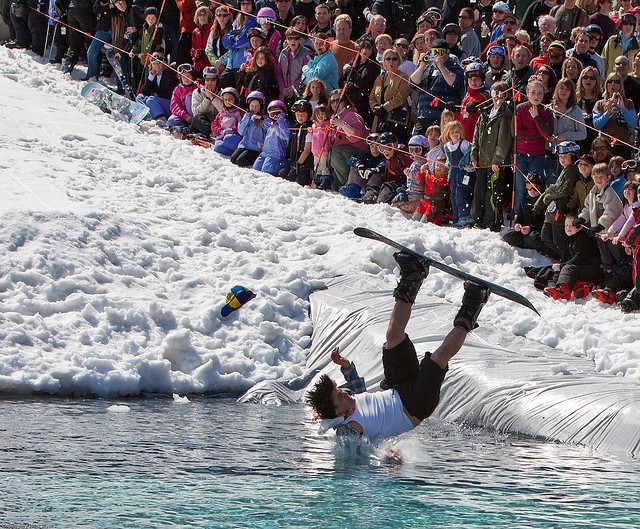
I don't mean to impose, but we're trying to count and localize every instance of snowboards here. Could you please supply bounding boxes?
[353,226,542,315]
[82,82,150,125]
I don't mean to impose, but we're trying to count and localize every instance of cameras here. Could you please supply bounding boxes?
[422,55,436,63]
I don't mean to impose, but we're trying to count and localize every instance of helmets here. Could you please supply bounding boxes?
[463,62,485,77]
[423,7,443,28]
[220,87,240,98]
[247,26,266,39]
[492,2,510,13]
[267,100,288,115]
[585,23,604,48]
[442,23,460,42]
[557,140,580,159]
[245,91,266,104]
[256,7,276,17]
[291,100,312,117]
[407,135,429,148]
[487,46,506,66]
[377,131,398,147]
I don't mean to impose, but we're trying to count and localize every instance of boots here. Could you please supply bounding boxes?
[392,250,429,302]
[454,280,491,332]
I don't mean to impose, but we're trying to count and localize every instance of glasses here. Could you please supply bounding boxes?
[607,78,620,83]
[386,56,398,60]
[203,67,219,75]
[365,135,380,145]
[197,13,208,16]
[408,144,423,154]
[268,109,285,119]
[622,14,636,22]
[539,69,550,75]
[458,15,470,20]
[429,49,447,56]
[594,146,607,151]
[177,63,193,73]
[494,9,504,14]
[525,182,541,190]
[248,27,266,36]
[256,17,276,25]
[396,43,408,48]
[330,98,345,103]
[465,69,483,76]
[379,140,398,153]
[490,88,508,98]
[425,9,442,20]
[588,31,601,39]
[291,103,310,111]
[621,158,638,169]
[615,60,628,65]
[204,78,217,81]
[583,74,596,80]
[287,35,300,40]
[552,144,580,154]
[444,24,462,33]
[504,19,515,25]
[486,45,507,57]
[216,13,229,17]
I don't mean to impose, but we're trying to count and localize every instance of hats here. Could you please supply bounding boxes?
[547,39,567,50]
[595,0,615,6]
[432,39,449,48]
[574,154,597,166]
[502,12,517,21]
[145,6,159,17]
[221,285,256,317]
[524,168,547,183]
[358,34,375,51]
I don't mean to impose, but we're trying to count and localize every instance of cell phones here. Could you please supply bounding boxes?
[612,93,619,106]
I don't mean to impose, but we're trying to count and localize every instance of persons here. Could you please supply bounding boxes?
[490,0,639,311]
[303,252,490,466]
[2,0,490,232]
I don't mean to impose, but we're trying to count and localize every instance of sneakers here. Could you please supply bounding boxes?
[357,190,377,204]
[591,288,616,304]
[574,280,594,300]
[544,284,572,301]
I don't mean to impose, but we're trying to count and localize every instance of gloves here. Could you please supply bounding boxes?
[572,217,586,228]
[466,99,484,114]
[354,159,367,180]
[362,165,380,179]
[444,99,461,112]
[372,104,387,118]
[559,205,571,217]
[586,223,605,237]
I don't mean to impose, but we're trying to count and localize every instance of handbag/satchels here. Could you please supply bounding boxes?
[386,106,410,126]
